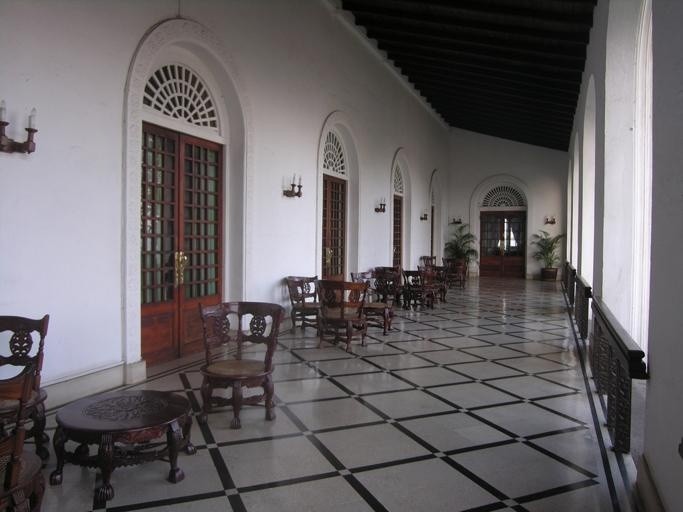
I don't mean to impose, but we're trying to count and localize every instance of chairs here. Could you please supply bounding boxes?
[280,256,467,349]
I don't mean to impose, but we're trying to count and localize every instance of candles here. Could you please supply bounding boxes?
[290,173,303,187]
[0,99,9,122]
[27,107,37,130]
[380,196,385,204]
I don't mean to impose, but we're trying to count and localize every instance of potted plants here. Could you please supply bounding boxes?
[442,224,479,277]
[529,228,565,281]
